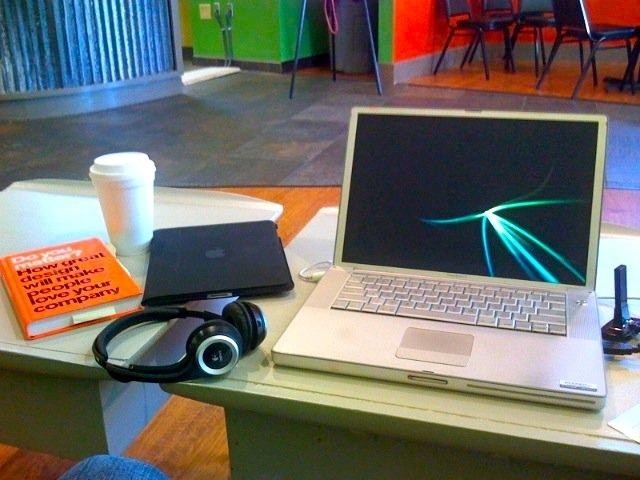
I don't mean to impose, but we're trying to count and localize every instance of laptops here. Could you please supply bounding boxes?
[142,220,293,303]
[271,105,607,410]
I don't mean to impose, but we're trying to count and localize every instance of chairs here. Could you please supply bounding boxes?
[434,1,640,101]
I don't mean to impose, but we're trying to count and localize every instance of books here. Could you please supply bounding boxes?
[0,236,145,342]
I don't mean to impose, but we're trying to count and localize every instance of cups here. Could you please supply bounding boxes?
[89,150,157,255]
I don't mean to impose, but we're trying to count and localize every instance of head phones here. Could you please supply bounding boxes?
[91,301,265,383]
